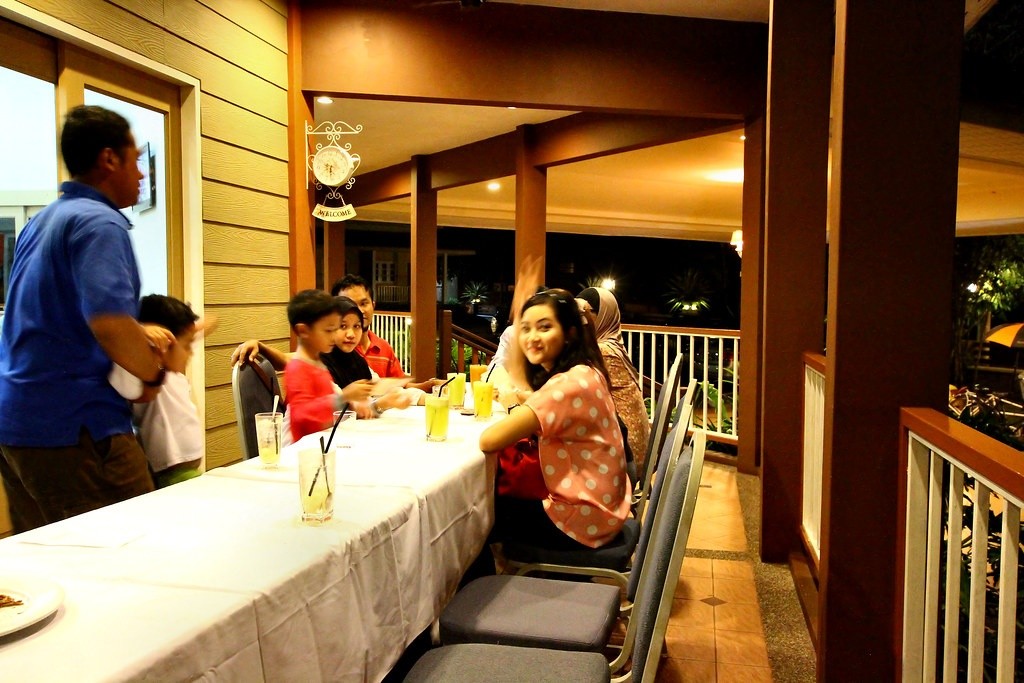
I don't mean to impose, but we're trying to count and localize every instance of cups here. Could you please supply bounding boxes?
[432,384,447,396]
[472,381,492,421]
[254,412,283,471]
[334,409,355,450]
[424,396,448,442]
[448,372,467,409]
[299,448,336,526]
[470,364,488,391]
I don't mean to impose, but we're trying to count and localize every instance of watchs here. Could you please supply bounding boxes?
[507,404,520,414]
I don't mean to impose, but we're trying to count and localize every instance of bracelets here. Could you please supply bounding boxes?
[372,400,383,414]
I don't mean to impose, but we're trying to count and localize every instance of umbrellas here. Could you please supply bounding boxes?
[984,323,1024,348]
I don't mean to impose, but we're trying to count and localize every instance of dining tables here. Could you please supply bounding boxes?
[0,380,500,683]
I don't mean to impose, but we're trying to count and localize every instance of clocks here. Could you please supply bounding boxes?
[305,120,364,223]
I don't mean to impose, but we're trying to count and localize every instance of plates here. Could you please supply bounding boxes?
[0,574,62,634]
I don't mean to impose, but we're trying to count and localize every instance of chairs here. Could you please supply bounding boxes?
[232,354,289,459]
[398,353,706,683]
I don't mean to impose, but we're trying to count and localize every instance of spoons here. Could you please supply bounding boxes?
[320,436,332,510]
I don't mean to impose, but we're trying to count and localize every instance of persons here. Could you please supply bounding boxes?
[286,290,375,443]
[131,295,205,490]
[230,274,449,394]
[317,295,412,419]
[476,286,652,564]
[1,104,179,535]
